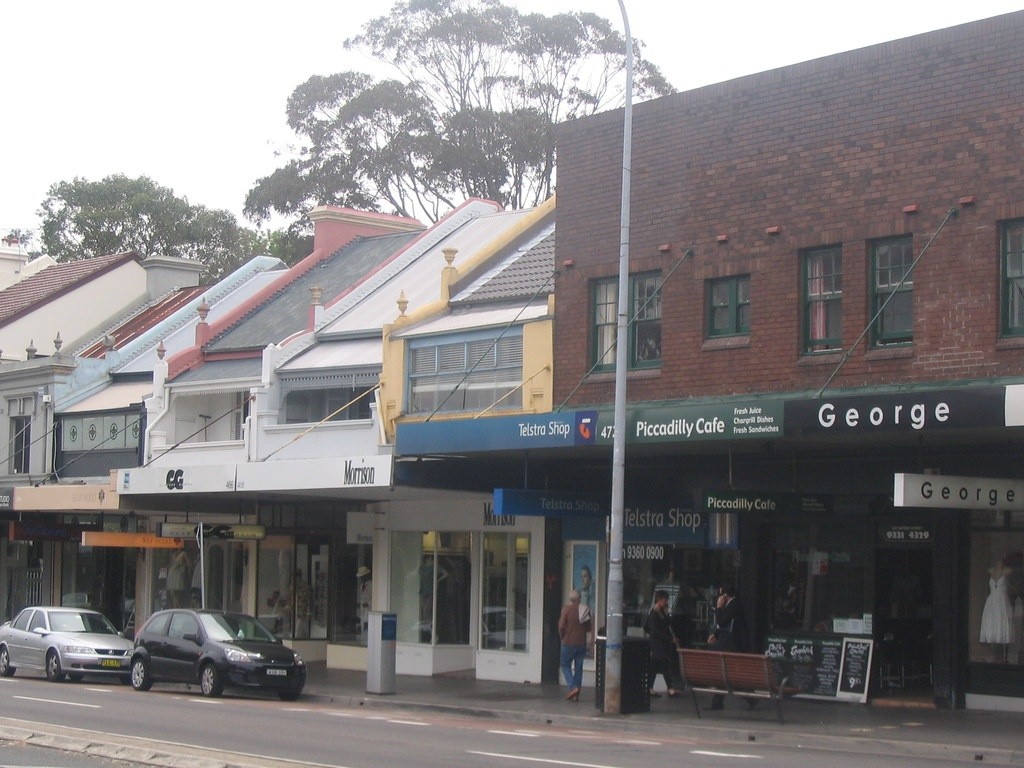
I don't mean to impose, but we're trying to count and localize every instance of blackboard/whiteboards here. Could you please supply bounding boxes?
[814,638,842,698]
[836,637,874,703]
[788,637,814,699]
[763,635,788,696]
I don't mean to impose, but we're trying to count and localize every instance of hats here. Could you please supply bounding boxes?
[356,566,371,577]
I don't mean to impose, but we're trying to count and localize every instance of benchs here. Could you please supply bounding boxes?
[677,648,800,725]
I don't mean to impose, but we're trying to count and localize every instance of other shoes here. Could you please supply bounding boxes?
[651,693,661,697]
[669,691,688,697]
[567,688,579,699]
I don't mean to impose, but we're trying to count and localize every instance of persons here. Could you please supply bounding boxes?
[560,591,592,701]
[191,553,202,609]
[165,552,192,608]
[702,584,759,710]
[580,565,592,606]
[419,557,448,643]
[287,568,313,639]
[643,590,680,696]
[356,567,372,645]
[980,559,1016,643]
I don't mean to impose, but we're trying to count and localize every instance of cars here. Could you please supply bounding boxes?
[0,605,135,683]
[130,608,306,701]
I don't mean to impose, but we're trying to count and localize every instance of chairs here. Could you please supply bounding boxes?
[878,637,933,688]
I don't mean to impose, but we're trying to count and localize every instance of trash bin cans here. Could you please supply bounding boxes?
[597,637,650,714]
